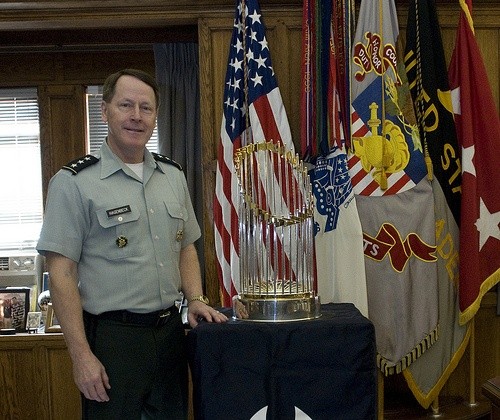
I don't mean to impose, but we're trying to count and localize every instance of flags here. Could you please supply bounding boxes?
[396,0,462,235]
[208,0,318,304]
[297,0,370,322]
[434,2,500,324]
[343,0,475,410]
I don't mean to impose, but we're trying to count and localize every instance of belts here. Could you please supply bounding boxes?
[82,305,178,328]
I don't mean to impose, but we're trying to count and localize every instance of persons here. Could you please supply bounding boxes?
[1,296,24,329]
[43,67,228,420]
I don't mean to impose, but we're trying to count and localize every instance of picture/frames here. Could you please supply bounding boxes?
[0,288,31,333]
[44,302,63,333]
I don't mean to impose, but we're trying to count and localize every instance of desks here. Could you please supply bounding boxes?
[0,331,82,420]
[186,303,380,420]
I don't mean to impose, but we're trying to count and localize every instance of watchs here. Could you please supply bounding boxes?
[187,294,209,304]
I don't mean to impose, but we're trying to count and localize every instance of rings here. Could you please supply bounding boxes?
[216,311,219,314]
